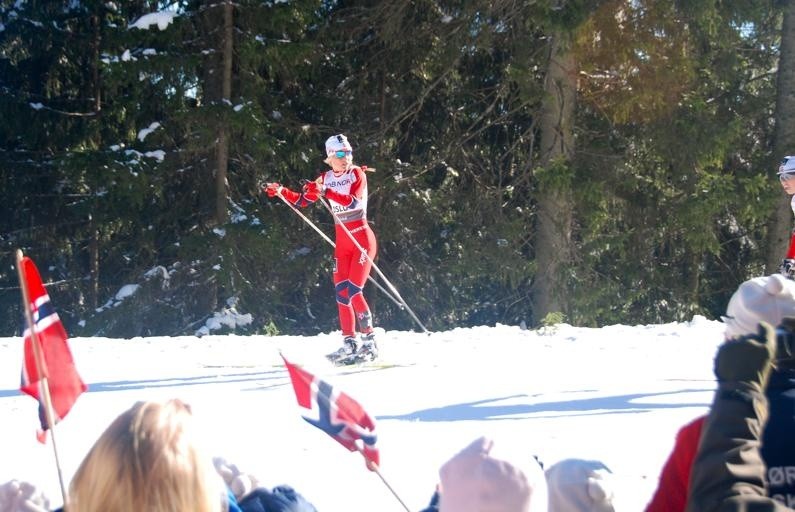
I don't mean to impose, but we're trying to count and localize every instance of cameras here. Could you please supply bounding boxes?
[775,329,795,362]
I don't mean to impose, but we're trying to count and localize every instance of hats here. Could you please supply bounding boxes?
[720,274,795,340]
[439,435,616,512]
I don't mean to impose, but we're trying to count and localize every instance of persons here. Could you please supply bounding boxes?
[417,437,532,512]
[48,398,316,512]
[545,458,620,512]
[685,318,795,512]
[641,274,795,512]
[262,133,379,362]
[0,478,48,512]
[776,155,795,282]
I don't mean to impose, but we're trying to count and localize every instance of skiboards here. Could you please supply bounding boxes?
[202,358,422,391]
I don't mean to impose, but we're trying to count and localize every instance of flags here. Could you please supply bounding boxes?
[283,360,380,469]
[17,256,88,444]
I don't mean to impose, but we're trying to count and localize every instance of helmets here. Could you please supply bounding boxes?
[326,134,352,155]
[776,155,795,175]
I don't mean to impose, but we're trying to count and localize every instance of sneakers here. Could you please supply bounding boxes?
[328,345,379,365]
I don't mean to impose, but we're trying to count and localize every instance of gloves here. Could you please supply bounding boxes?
[303,181,326,196]
[264,183,282,197]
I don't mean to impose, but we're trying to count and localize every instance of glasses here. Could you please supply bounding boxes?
[336,151,351,158]
[780,174,792,180]
